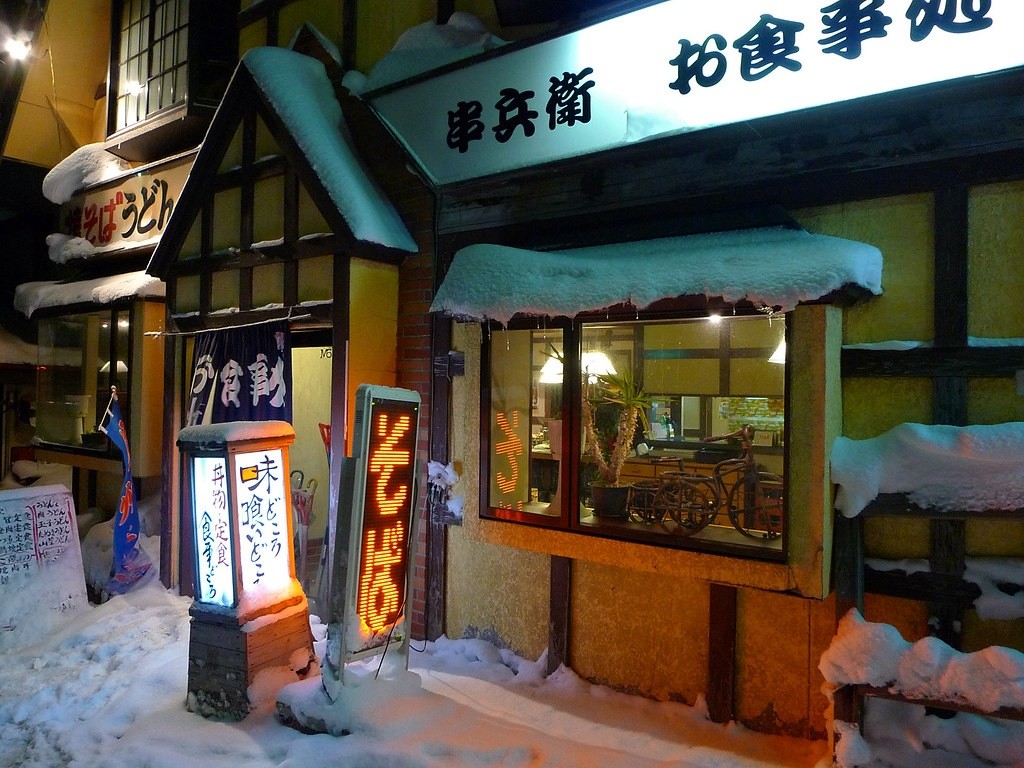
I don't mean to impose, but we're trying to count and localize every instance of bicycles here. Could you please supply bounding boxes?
[658,423,784,541]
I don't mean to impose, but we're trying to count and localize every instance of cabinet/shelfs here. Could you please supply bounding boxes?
[619,448,747,531]
